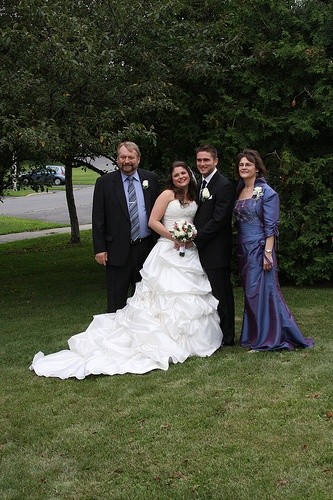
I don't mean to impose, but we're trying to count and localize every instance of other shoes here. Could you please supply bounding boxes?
[222,336,235,346]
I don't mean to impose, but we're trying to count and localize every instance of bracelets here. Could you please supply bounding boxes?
[265,250,272,252]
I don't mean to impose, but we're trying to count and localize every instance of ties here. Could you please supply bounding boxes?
[199,180,207,203]
[128,176,141,241]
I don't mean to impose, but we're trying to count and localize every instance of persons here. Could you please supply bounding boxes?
[232,149,313,353]
[191,145,235,346]
[30,161,224,379]
[92,142,159,313]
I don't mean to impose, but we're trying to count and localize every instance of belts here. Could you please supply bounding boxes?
[130,236,148,244]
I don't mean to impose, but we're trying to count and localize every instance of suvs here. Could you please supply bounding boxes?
[18,164,65,186]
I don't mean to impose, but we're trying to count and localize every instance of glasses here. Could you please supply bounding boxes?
[238,163,253,168]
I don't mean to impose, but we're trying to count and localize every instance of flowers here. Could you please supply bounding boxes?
[169,219,198,258]
[142,180,150,193]
[252,186,264,201]
[201,188,213,200]
